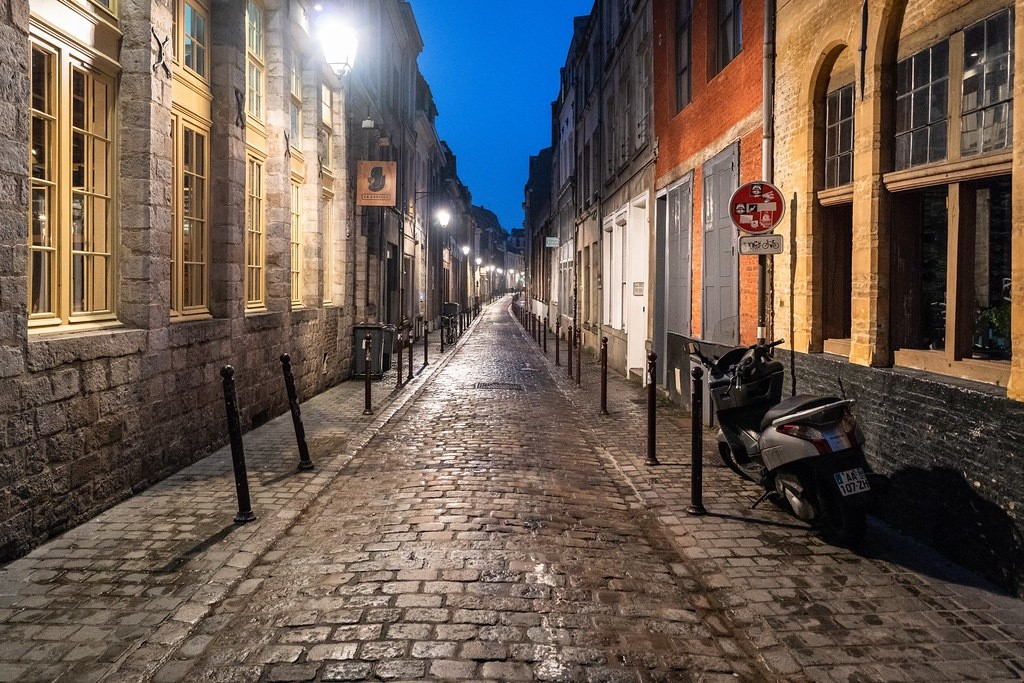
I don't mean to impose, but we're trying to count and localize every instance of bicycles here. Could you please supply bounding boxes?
[442,315,458,344]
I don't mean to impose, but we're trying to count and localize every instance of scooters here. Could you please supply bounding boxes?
[685,314,876,546]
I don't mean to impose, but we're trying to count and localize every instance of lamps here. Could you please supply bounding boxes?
[415,180,450,228]
[320,35,359,92]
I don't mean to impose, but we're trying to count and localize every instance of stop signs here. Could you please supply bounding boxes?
[727,181,785,234]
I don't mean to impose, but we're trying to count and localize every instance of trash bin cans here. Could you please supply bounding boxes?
[353,322,385,380]
[382,324,395,371]
[443,301,459,319]
